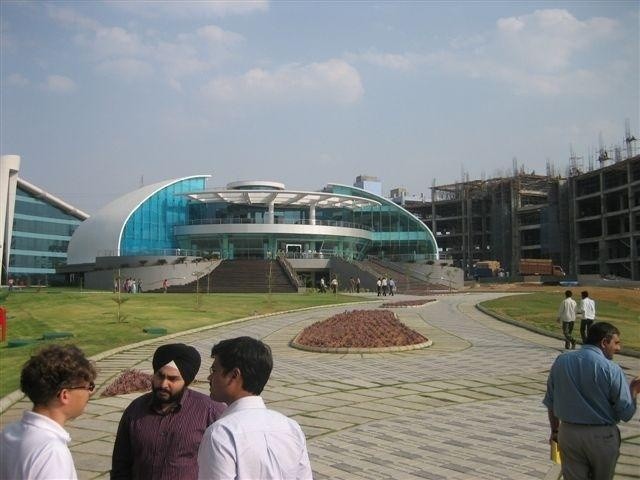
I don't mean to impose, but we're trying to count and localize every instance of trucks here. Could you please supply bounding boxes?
[476,261,506,277]
[518,256,565,280]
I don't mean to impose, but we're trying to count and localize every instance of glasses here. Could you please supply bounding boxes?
[57,382,95,396]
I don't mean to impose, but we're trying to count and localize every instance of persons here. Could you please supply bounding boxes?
[197,335,314,480]
[375,276,398,297]
[108,342,227,480]
[163,278,169,293]
[1,343,97,480]
[542,322,640,480]
[321,276,327,293]
[349,277,355,292]
[576,290,597,344]
[8,276,14,292]
[317,277,325,293]
[267,248,323,261]
[122,276,143,293]
[555,290,578,349]
[355,277,361,293]
[330,277,339,294]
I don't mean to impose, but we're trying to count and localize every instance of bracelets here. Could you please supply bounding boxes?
[552,429,559,434]
